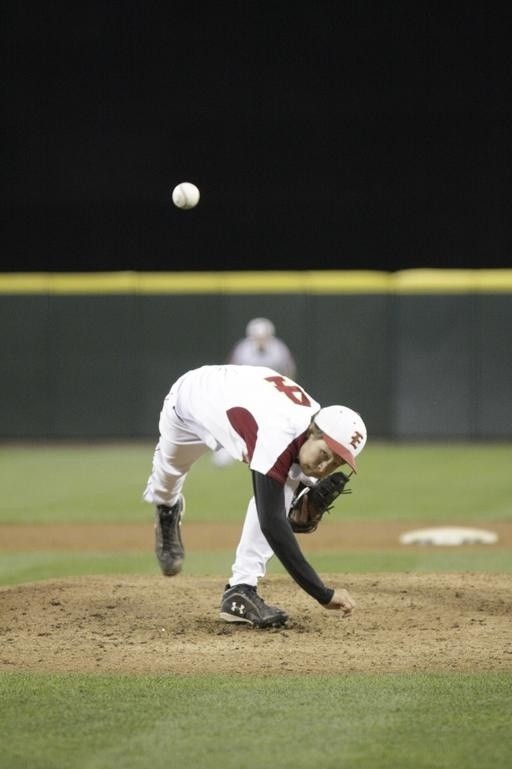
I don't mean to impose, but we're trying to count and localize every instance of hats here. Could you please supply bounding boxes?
[314,405,367,475]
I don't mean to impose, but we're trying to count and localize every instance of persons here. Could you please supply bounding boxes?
[229,316,298,381]
[141,362,370,631]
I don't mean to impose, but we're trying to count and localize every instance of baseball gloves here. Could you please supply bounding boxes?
[288,472,349,532]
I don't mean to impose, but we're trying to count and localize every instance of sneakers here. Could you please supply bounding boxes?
[218,584,287,628]
[153,494,187,577]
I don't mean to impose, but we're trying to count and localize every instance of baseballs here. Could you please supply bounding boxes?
[174,183,201,209]
